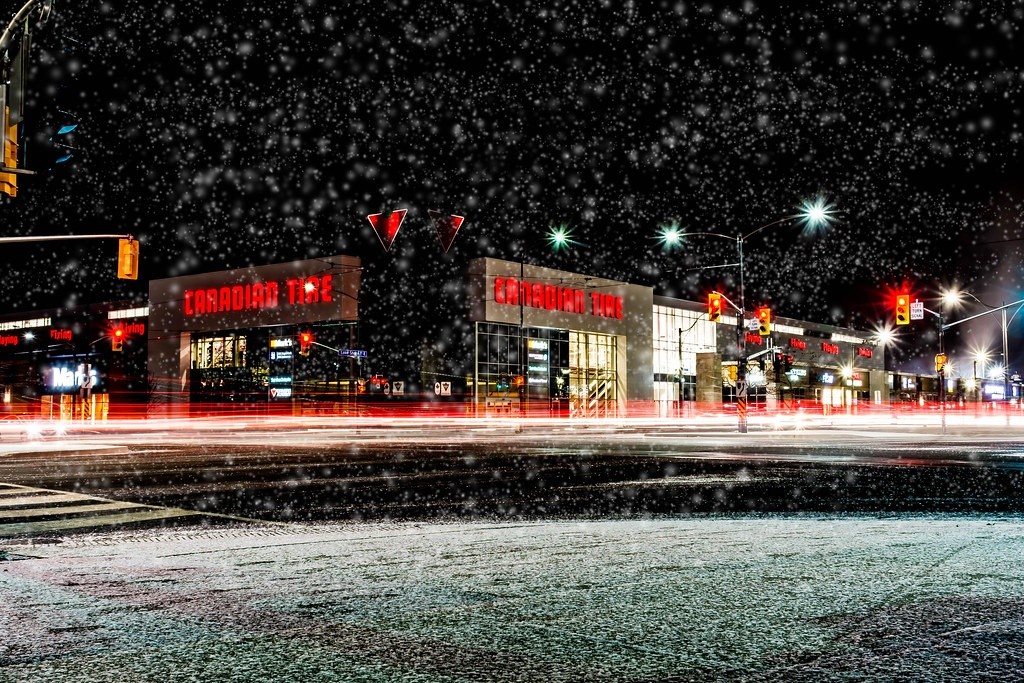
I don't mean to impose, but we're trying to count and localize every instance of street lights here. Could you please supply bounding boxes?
[947,292,1024,424]
[25,332,77,404]
[973,351,991,413]
[304,283,364,409]
[655,205,829,433]
[851,333,894,412]
[519,228,568,412]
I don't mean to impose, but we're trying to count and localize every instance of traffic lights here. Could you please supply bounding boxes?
[504,373,510,389]
[786,355,792,372]
[301,333,310,356]
[726,366,737,380]
[737,358,747,379]
[757,355,769,370]
[895,295,910,327]
[758,308,770,336]
[112,329,123,352]
[496,372,502,390]
[935,355,946,374]
[515,377,523,385]
[709,292,721,322]
[775,352,782,369]
[10,15,85,175]
